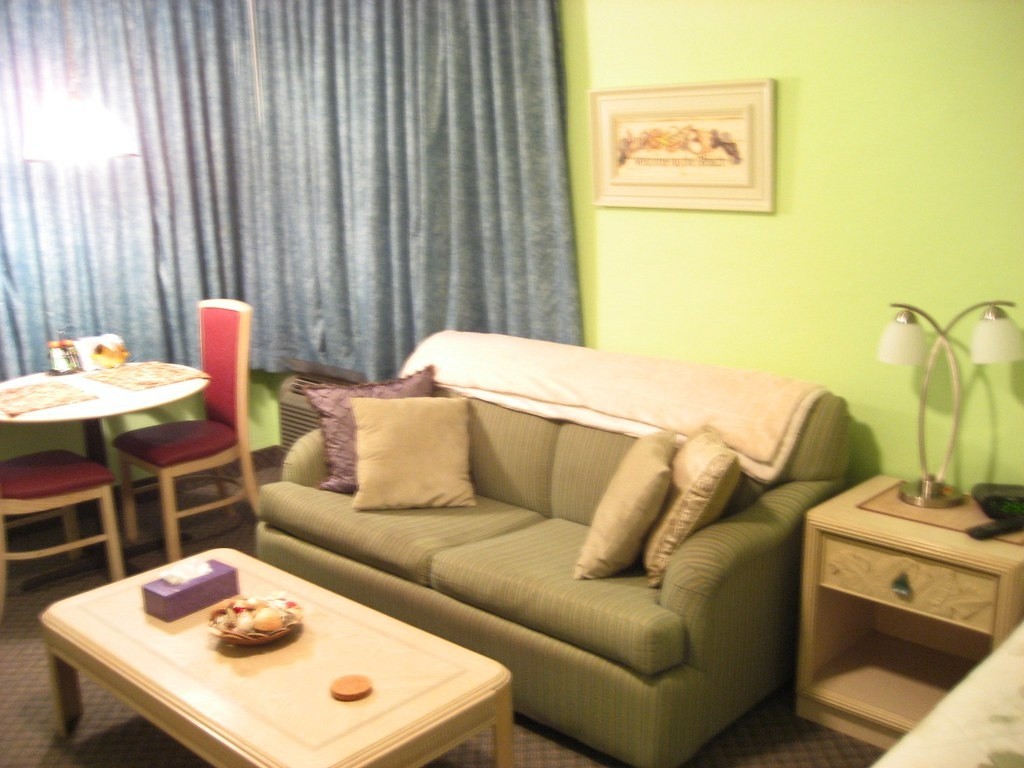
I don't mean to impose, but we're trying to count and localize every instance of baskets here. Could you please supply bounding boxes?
[207,597,304,646]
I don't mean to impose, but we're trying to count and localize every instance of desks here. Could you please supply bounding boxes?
[0,360,209,589]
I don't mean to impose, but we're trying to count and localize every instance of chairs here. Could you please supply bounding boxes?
[111,299,261,561]
[0,450,128,621]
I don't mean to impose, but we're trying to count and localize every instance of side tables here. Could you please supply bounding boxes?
[795,473,1024,750]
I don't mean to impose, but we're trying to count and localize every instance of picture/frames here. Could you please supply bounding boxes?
[591,78,777,212]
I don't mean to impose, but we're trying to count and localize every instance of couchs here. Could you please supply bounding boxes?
[255,330,856,768]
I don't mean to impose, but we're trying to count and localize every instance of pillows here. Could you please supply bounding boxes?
[304,365,437,493]
[574,431,677,580]
[643,425,742,589]
[349,397,477,508]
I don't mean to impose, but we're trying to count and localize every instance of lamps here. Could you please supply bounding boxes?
[876,300,1024,513]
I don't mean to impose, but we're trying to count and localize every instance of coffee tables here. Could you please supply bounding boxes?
[39,545,514,768]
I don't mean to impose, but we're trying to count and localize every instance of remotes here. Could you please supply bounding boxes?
[968,518,1024,540]
